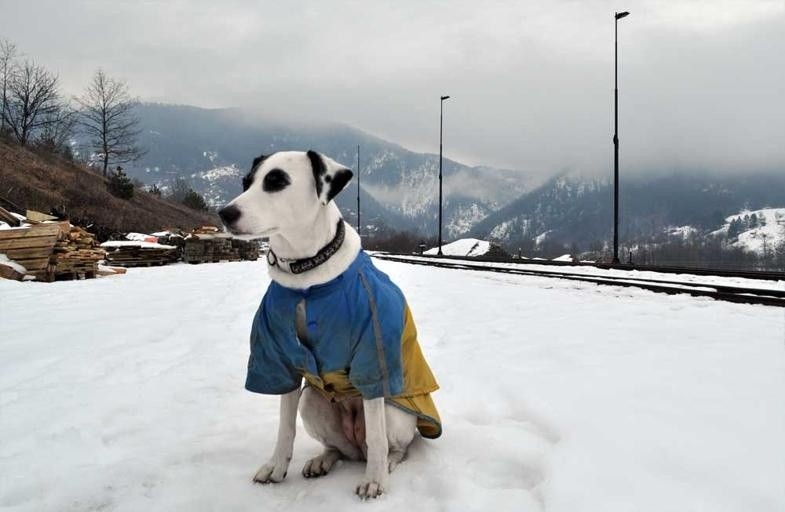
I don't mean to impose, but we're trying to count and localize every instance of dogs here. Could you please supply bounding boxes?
[216,149,443,502]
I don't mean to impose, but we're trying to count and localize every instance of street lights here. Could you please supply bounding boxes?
[438,95,451,257]
[613,11,631,264]
